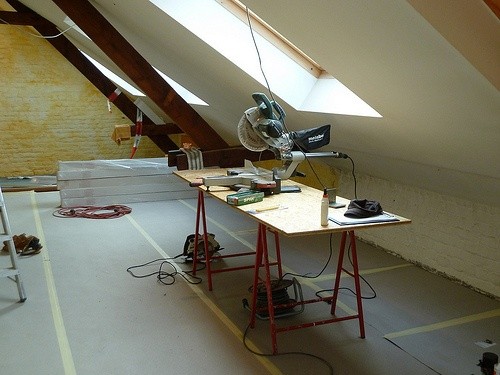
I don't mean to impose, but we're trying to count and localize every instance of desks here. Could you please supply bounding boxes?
[172,167,413,359]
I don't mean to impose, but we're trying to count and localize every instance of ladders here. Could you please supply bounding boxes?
[0,186,27,303]
